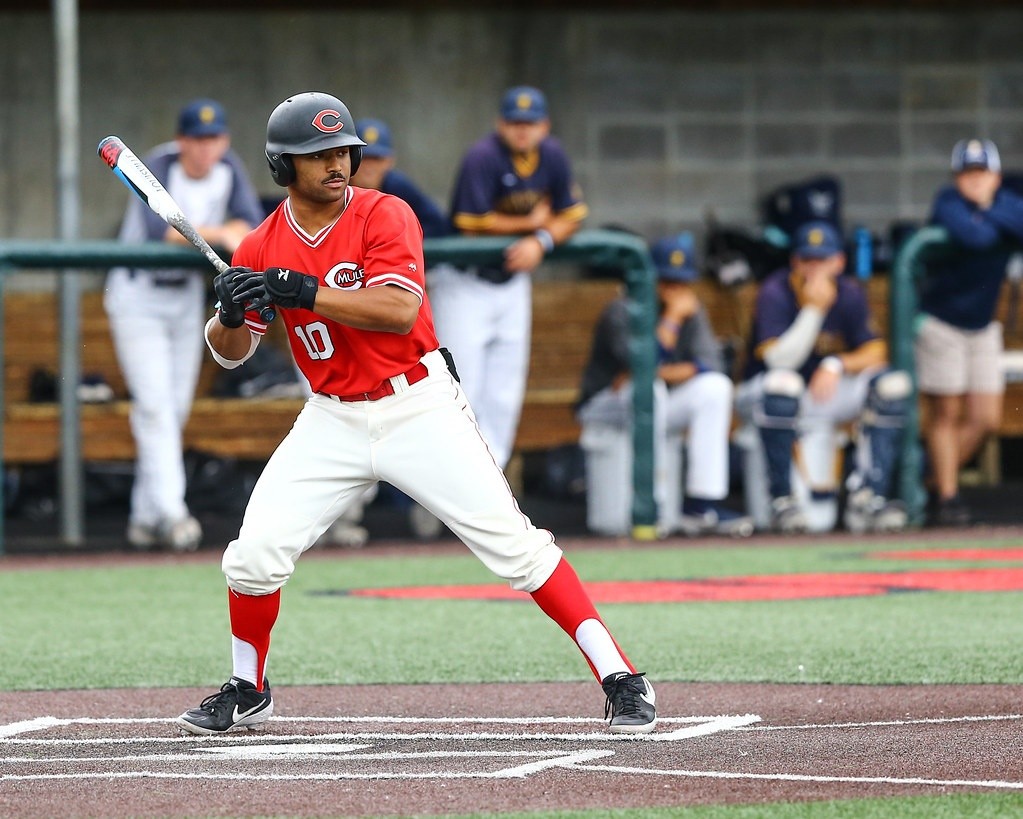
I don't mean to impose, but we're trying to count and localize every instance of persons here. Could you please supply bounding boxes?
[910,130,1023,528]
[412,84,592,536]
[177,90,656,733]
[314,117,451,547]
[102,94,264,553]
[568,228,754,534]
[731,220,915,536]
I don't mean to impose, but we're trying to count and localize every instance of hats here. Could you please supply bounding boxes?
[177,100,227,137]
[649,237,698,284]
[353,115,391,161]
[499,86,547,125]
[795,222,843,262]
[949,133,1000,172]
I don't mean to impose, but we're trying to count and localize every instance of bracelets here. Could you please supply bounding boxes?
[819,354,844,376]
[536,229,554,253]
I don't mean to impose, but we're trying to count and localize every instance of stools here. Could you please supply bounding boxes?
[735,427,837,531]
[580,429,681,533]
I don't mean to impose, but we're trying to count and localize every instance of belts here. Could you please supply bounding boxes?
[320,360,428,405]
[151,276,190,289]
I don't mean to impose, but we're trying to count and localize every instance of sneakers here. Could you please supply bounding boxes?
[600,668,659,737]
[178,673,276,734]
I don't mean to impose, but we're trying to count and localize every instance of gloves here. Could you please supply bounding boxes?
[213,263,256,330]
[230,265,321,313]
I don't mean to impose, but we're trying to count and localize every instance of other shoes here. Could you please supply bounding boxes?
[327,517,368,546]
[411,508,443,541]
[938,489,980,526]
[845,492,904,534]
[678,506,753,539]
[126,514,203,551]
[772,497,807,532]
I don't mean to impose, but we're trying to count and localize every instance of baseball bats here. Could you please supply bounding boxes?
[94,134,277,324]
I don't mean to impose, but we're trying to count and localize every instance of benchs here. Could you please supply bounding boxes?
[1,277,1023,488]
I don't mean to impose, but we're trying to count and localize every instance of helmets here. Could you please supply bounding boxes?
[265,91,368,188]
